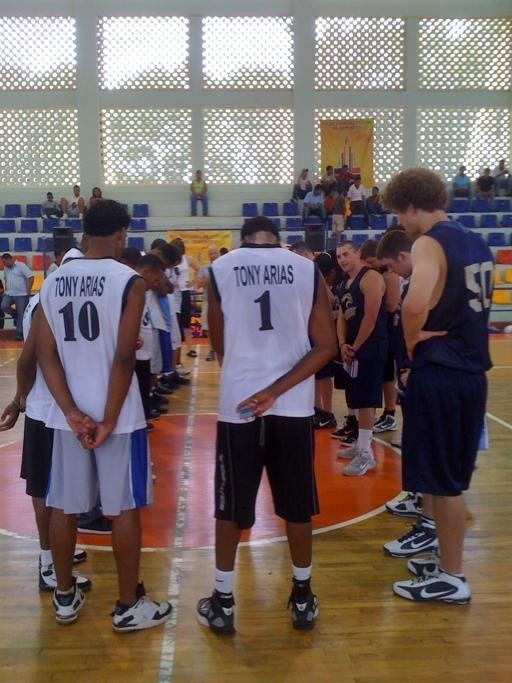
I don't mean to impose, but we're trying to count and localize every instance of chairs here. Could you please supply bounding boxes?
[1,197,150,308]
[240,190,511,305]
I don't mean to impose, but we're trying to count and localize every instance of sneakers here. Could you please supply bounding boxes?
[404,555,443,579]
[389,436,403,449]
[287,585,322,631]
[37,567,94,594]
[329,415,361,446]
[384,490,425,517]
[390,571,473,607]
[372,411,398,433]
[50,575,89,625]
[334,440,378,478]
[186,349,198,357]
[141,361,192,434]
[110,594,174,634]
[311,404,339,431]
[76,508,115,537]
[380,517,440,559]
[205,350,217,361]
[196,594,238,640]
[36,545,89,569]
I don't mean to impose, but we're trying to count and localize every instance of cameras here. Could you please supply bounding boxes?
[240,403,254,421]
